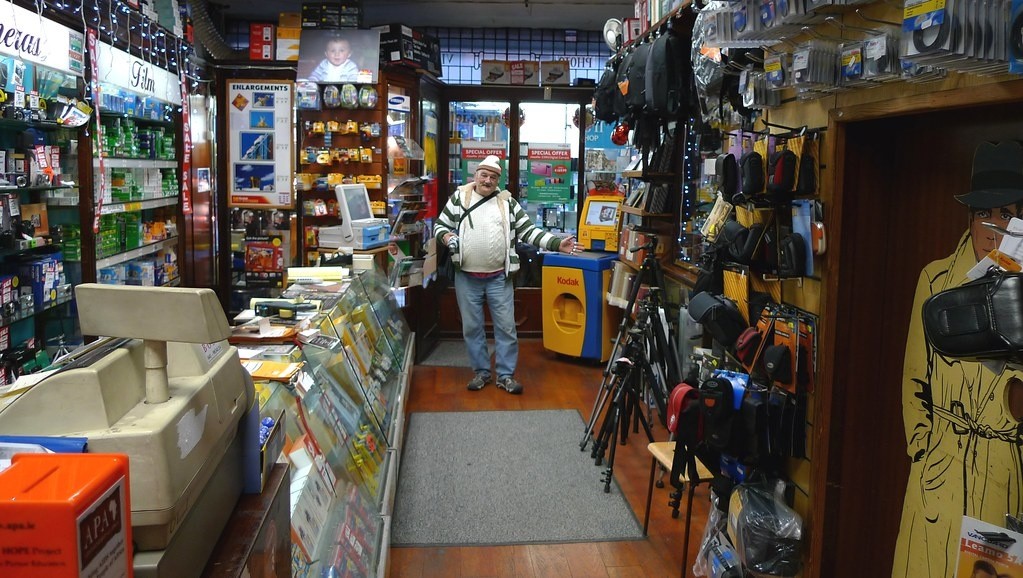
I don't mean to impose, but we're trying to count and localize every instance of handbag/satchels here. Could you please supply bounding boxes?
[666,145,807,493]
[429,243,454,293]
[921,272,1023,359]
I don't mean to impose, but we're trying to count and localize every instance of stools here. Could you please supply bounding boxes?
[643,441,715,578]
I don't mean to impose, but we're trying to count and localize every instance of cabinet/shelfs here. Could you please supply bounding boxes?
[316,247,390,277]
[0,51,96,387]
[618,171,674,321]
[197,462,293,578]
[230,256,415,578]
[91,78,186,339]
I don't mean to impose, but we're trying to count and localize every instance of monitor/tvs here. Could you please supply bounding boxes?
[335,184,374,227]
[584,200,618,226]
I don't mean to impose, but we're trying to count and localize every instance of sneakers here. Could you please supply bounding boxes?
[495,375,522,394]
[467,374,492,391]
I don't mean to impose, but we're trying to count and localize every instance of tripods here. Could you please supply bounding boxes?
[580,234,684,493]
[46,332,74,366]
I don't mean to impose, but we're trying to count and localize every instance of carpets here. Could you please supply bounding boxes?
[387,408,648,549]
[419,341,495,367]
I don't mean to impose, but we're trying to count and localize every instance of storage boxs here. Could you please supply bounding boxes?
[127,0,194,46]
[249,13,302,60]
[32,260,59,309]
[302,1,362,30]
[481,60,540,86]
[369,23,442,76]
[540,61,571,85]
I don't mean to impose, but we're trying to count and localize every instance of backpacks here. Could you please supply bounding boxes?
[592,30,696,121]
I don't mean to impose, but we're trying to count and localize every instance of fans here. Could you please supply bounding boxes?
[603,17,621,53]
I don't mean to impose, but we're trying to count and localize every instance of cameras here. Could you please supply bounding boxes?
[0,294,35,318]
[56,283,71,298]
[4,105,25,122]
[7,172,30,188]
[35,174,50,187]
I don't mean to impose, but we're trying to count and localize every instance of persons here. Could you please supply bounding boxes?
[307,35,359,83]
[433,154,585,394]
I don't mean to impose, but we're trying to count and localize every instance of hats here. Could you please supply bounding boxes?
[475,155,501,176]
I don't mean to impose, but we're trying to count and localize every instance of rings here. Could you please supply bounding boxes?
[570,241,577,255]
